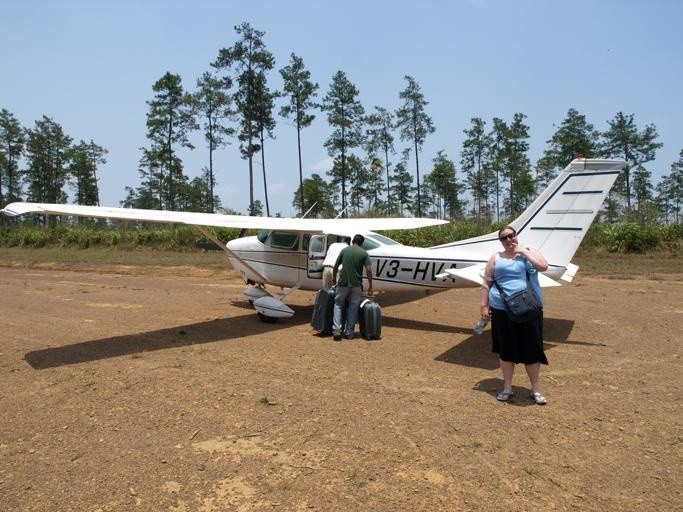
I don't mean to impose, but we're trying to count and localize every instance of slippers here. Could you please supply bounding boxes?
[496,389,513,403]
[530,387,547,405]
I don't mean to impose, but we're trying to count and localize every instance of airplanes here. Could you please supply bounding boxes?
[0,154,627,322]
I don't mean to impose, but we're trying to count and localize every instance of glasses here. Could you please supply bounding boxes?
[498,230,516,244]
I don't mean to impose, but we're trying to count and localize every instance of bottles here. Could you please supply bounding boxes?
[473,309,493,336]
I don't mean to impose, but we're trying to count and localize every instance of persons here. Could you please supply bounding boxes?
[331,234,374,340]
[479,225,549,405]
[321,237,351,288]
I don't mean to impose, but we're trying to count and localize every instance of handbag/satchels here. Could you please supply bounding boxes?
[502,284,543,324]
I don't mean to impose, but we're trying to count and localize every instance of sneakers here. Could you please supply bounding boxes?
[332,334,356,341]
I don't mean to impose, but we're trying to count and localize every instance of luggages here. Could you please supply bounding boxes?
[308,285,336,336]
[359,297,383,340]
